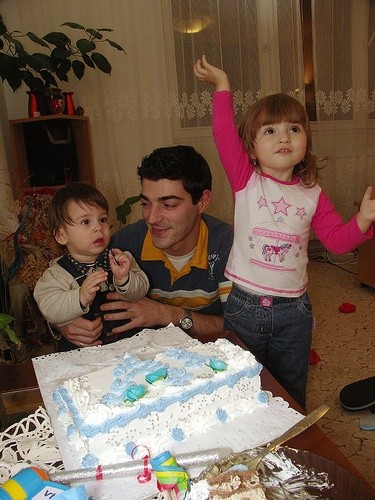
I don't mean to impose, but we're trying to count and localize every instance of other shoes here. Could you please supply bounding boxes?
[340,376,375,414]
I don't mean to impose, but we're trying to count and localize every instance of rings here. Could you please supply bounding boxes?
[79,343,84,347]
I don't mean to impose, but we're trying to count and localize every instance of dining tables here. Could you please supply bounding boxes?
[0,326,375,500]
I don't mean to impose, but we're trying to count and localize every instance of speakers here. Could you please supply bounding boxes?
[22,119,80,186]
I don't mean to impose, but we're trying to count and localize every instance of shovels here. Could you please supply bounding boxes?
[242,403,331,471]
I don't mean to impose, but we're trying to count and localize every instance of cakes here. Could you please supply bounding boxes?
[188,464,268,500]
[52,339,269,469]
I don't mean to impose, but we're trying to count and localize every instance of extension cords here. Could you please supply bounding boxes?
[322,251,355,261]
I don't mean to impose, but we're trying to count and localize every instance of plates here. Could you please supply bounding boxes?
[183,446,375,500]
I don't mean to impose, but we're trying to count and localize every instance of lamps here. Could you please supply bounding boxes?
[173,13,210,34]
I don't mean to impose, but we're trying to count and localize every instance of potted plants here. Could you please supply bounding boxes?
[0,14,127,118]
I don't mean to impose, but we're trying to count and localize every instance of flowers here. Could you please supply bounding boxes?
[208,360,226,373]
[122,384,147,401]
[146,367,167,384]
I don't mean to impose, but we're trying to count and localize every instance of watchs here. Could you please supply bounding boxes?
[179,309,193,334]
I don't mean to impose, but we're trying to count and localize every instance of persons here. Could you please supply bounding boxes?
[32,183,150,352]
[47,145,233,347]
[194,55,375,413]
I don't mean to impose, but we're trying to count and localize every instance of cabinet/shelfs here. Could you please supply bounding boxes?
[8,115,95,226]
